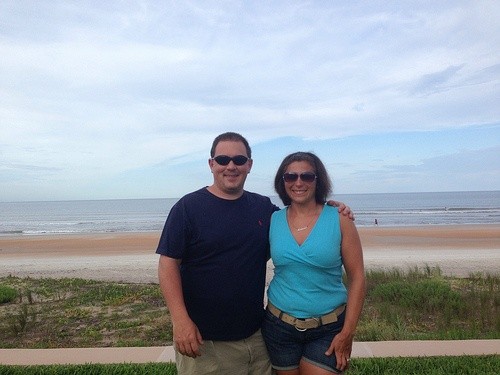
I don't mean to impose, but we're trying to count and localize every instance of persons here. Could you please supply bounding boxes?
[155,132,355,375]
[261,152,365,375]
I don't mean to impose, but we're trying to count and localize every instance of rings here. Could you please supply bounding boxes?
[346,358,351,361]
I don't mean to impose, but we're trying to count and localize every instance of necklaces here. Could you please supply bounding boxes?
[289,205,318,231]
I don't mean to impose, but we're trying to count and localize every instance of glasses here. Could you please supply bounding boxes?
[211,155,249,166]
[283,172,317,182]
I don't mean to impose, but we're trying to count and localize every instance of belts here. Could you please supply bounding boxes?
[268,303,346,332]
